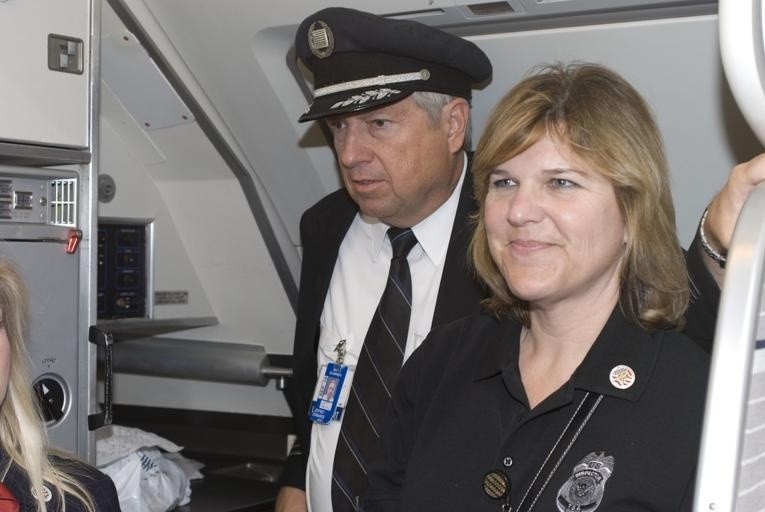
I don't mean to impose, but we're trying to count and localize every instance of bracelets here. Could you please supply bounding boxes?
[698,204,731,266]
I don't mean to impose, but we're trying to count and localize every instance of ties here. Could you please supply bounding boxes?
[331,226,418,512]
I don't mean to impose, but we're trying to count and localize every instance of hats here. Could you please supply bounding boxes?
[295,7,492,123]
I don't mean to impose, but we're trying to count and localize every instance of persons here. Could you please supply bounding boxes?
[270,5,764,512]
[0,267,123,511]
[342,63,715,512]
